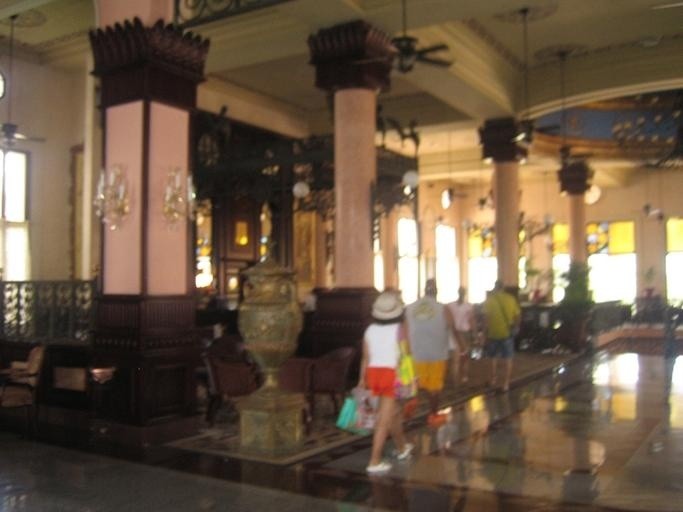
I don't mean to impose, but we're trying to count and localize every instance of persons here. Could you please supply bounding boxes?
[351,277,523,473]
[367,391,524,512]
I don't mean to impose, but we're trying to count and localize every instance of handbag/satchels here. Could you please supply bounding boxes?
[399,343,415,386]
[335,387,370,437]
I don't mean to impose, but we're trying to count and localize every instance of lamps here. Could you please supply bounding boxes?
[161,165,199,225]
[91,163,133,233]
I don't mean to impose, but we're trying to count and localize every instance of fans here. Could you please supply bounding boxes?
[353,0,452,75]
[1,0,49,150]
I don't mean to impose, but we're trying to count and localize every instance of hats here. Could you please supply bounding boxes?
[369,292,405,320]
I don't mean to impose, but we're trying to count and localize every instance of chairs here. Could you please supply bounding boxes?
[0,344,45,440]
[207,333,265,424]
[276,344,357,421]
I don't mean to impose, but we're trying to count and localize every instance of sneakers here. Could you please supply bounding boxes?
[427,414,448,424]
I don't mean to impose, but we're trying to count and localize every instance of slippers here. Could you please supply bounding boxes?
[397,442,414,459]
[365,460,392,471]
